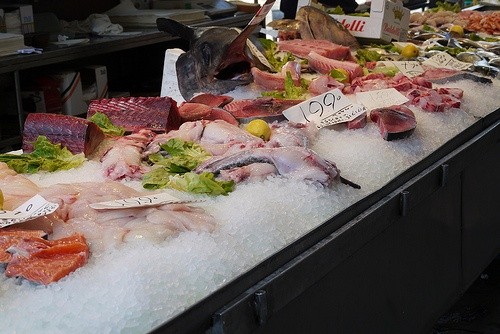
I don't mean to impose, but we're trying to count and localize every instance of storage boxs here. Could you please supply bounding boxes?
[295,0,410,44]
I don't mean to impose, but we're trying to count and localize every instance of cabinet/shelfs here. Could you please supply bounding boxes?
[0,0,259,149]
